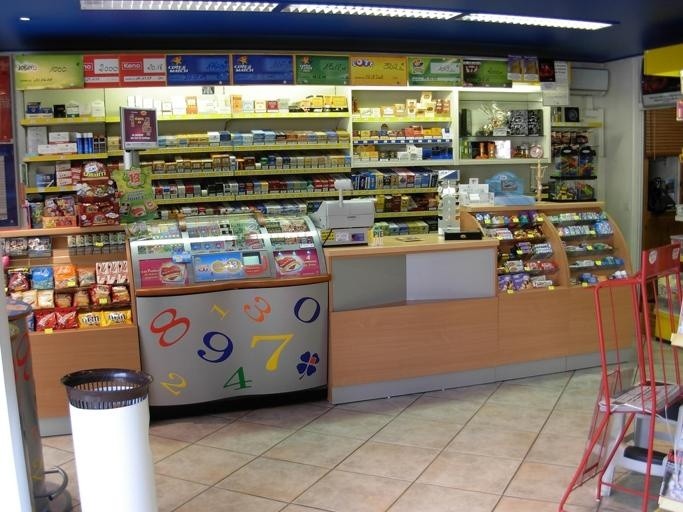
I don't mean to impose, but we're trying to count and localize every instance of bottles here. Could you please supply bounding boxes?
[459,137,473,159]
[368,225,384,248]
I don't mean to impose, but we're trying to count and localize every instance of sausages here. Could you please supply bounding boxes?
[161,267,182,275]
[280,258,296,267]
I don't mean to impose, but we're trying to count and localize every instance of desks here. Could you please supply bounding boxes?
[317,230,502,408]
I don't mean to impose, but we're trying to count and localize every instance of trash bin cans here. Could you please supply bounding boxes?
[60,368,158,512]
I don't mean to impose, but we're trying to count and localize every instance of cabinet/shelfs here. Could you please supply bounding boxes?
[18,106,351,214]
[346,84,553,205]
[127,216,329,421]
[458,202,568,384]
[535,202,636,371]
[0,219,145,438]
[548,103,607,204]
